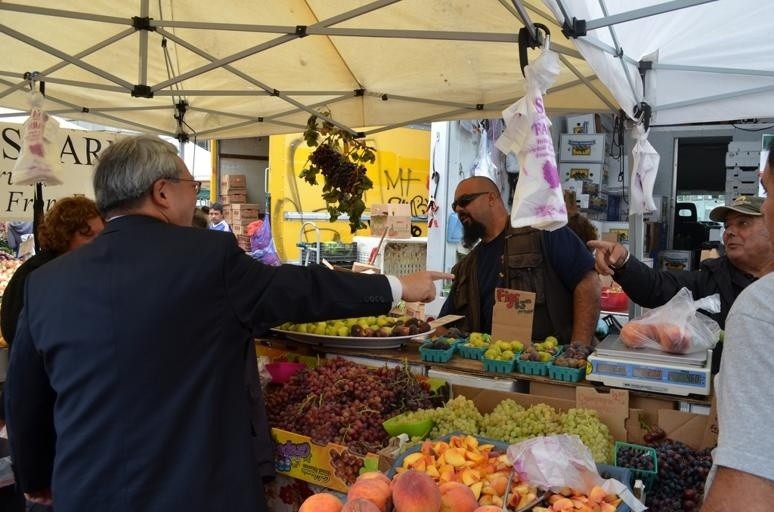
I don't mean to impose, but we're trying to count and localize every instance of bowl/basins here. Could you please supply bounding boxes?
[600,286,629,312]
[383,412,434,442]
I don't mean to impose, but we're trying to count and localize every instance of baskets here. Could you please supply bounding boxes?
[354,235,427,276]
[298,242,355,264]
[615,440,660,491]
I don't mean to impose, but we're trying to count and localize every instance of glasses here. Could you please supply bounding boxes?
[451,191,490,211]
[152,176,203,195]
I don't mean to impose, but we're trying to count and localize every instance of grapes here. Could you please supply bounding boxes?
[617,432,712,512]
[265,356,610,465]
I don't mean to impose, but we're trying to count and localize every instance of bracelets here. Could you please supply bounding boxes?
[608,248,630,271]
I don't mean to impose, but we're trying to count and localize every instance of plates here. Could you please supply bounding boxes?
[268,326,438,350]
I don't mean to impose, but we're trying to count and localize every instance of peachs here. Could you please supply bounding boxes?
[621,321,691,353]
[0,257,23,297]
[298,470,502,512]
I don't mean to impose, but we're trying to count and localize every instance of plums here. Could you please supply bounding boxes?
[280,315,430,336]
[422,330,592,368]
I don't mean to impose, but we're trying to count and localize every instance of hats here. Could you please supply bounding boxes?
[708,193,767,222]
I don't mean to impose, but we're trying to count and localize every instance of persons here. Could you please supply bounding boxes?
[192,202,234,232]
[584,194,774,377]
[2,133,457,512]
[561,188,598,253]
[697,136,773,510]
[432,174,602,359]
[0,194,102,361]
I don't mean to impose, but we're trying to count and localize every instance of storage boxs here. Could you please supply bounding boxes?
[220,174,260,252]
[558,111,691,271]
[269,334,655,512]
[370,204,411,240]
